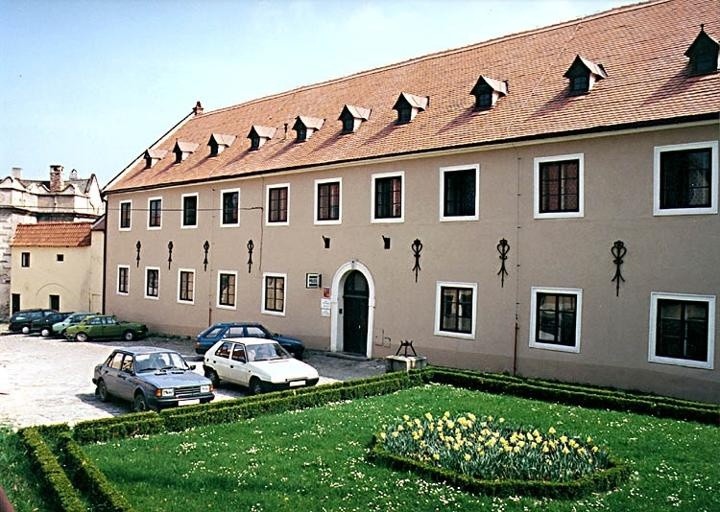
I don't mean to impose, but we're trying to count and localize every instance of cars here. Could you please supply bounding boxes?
[203,337,319,394]
[195,322,305,359]
[92,345,214,411]
[9,309,148,343]
